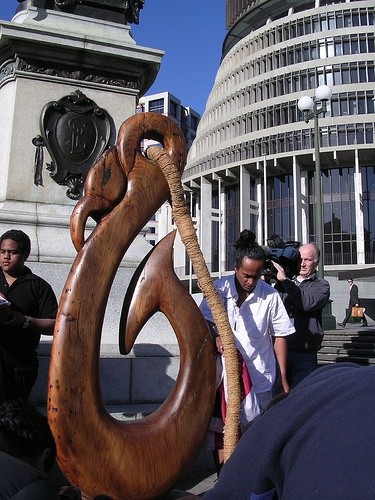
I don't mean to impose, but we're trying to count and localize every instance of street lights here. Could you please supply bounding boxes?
[298,85,332,331]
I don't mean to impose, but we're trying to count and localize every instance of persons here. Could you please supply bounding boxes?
[0,400,58,500]
[181,362,375,500]
[0,230,58,400]
[271,244,330,398]
[199,229,296,478]
[337,278,368,327]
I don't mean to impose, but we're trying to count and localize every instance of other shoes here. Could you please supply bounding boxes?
[337,323,345,328]
[362,324,367,327]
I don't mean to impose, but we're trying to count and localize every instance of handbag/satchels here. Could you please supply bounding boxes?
[351,307,366,318]
